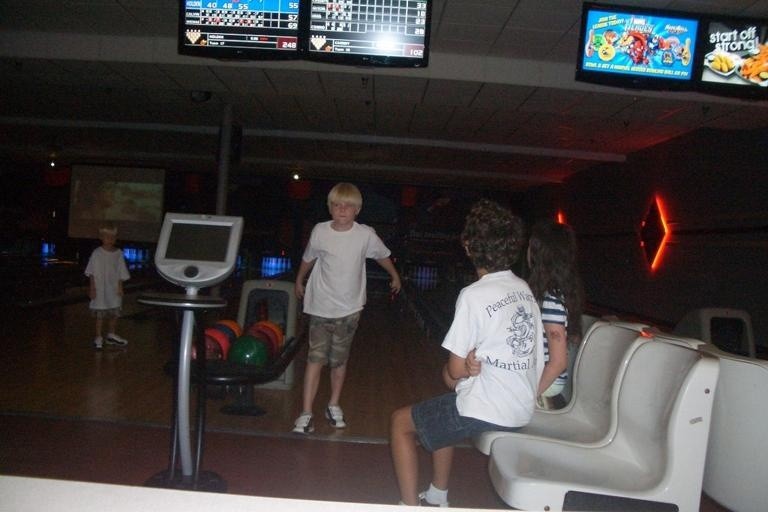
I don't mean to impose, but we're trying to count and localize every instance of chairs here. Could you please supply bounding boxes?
[470,312,768,512]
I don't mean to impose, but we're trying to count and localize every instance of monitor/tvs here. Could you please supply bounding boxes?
[576,1,706,91]
[303,0,434,69]
[153,212,244,283]
[697,12,768,102]
[178,0,304,60]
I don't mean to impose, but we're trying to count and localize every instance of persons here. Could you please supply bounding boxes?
[525,218,585,401]
[84,221,130,351]
[388,196,546,508]
[292,181,402,436]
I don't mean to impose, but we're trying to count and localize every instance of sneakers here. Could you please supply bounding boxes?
[291,413,316,435]
[107,333,128,347]
[398,493,449,507]
[324,403,347,430]
[94,337,104,349]
